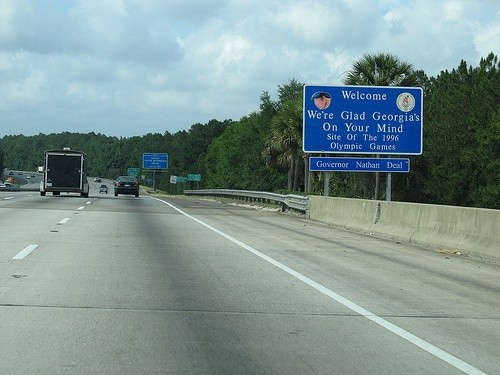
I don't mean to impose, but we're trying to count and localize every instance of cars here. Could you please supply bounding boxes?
[113,175,140,197]
[9,171,14,175]
[93,177,101,182]
[18,172,23,175]
[26,174,36,179]
[99,184,108,194]
[0,176,15,189]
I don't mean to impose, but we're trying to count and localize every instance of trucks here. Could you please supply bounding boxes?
[37,147,90,198]
